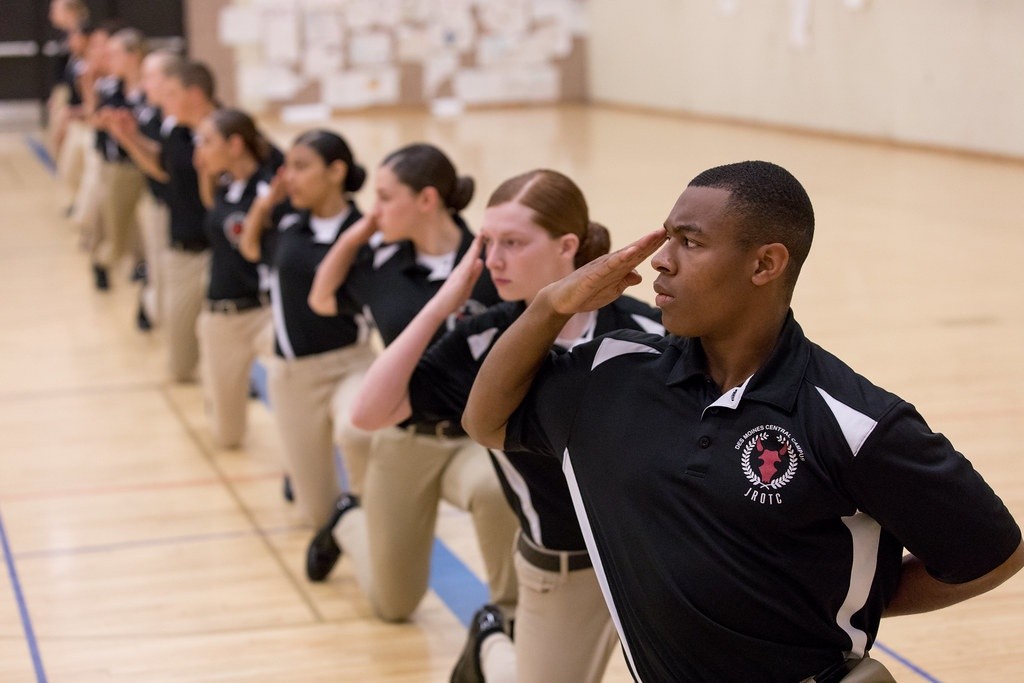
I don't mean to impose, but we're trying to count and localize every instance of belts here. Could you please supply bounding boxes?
[518,536,592,572]
[814,650,869,683]
[398,416,468,436]
[238,291,270,310]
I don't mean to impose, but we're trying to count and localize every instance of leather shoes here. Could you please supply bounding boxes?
[450,605,505,683]
[306,493,358,581]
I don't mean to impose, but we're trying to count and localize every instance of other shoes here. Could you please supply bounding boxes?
[97,270,108,287]
[285,477,293,500]
[140,311,150,329]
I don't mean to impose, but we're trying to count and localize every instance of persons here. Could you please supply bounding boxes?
[459,159,1024,683]
[348,168,672,683]
[307,143,515,645]
[44,0,374,531]
